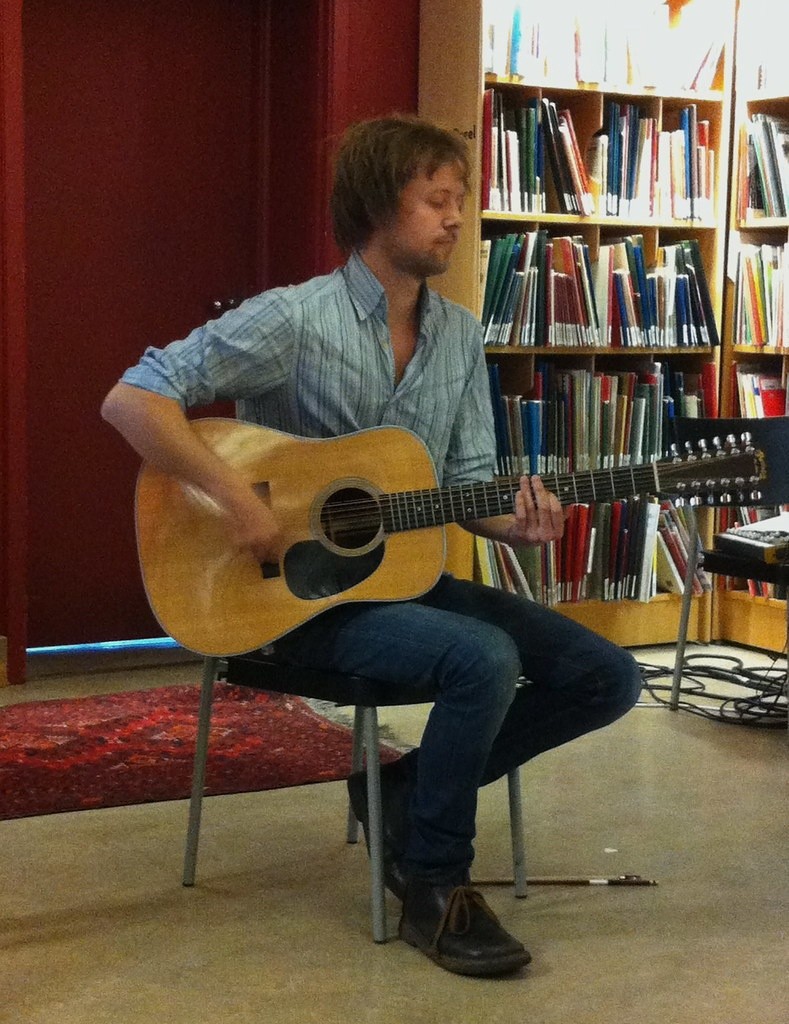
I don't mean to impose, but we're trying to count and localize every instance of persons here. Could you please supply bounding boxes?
[101,118,643,978]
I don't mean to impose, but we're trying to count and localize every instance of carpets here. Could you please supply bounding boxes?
[0,683,401,822]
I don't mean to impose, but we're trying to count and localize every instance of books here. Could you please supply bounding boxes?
[473,1,789,607]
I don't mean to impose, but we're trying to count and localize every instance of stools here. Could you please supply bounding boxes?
[181,658,527,943]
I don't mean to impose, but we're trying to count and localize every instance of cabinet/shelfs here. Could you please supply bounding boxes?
[418,0,789,661]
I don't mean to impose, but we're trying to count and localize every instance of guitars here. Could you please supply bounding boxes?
[134,415,770,657]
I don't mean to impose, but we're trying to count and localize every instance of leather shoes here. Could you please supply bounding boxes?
[348,759,413,902]
[398,881,533,977]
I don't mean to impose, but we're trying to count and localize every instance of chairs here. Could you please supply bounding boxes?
[670,416,789,712]
